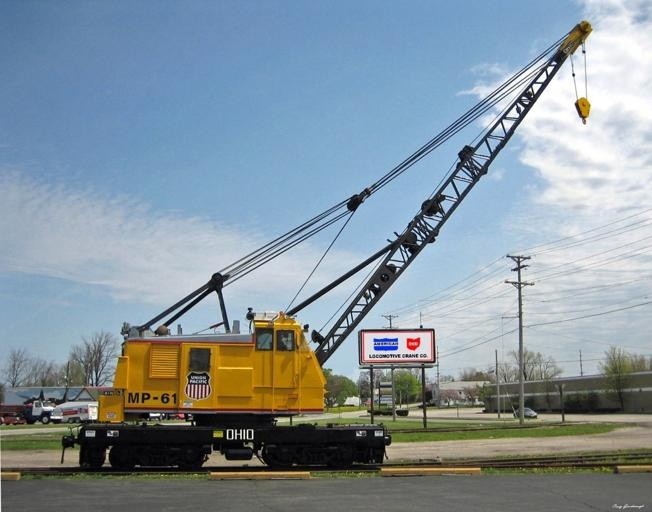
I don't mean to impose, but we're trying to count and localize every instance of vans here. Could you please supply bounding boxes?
[148,412,163,422]
[168,413,185,420]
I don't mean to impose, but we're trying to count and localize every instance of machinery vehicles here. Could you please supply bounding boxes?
[59,19,592,472]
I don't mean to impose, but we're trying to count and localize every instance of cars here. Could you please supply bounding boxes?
[513,407,538,419]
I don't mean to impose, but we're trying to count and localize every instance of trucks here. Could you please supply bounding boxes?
[0,399,57,425]
[49,401,99,423]
